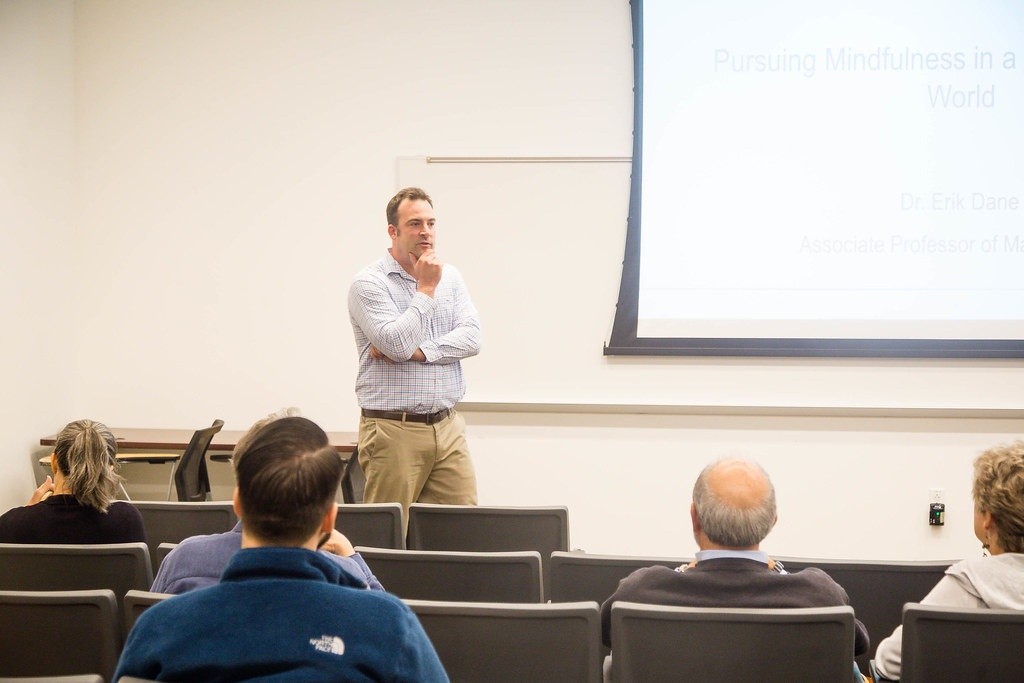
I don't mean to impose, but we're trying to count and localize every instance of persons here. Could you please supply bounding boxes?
[600,455,870,657]
[347,187,482,540]
[112,407,451,683]
[874,439,1024,680]
[0,419,146,544]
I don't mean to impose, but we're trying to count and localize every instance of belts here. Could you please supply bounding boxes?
[362,408,452,425]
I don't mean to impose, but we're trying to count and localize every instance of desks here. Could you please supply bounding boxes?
[210,454,351,486]
[38,453,181,503]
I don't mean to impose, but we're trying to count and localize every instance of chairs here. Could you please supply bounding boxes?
[549,551,694,658]
[339,444,367,506]
[610,600,857,683]
[901,601,1024,683]
[353,544,545,605]
[111,499,237,564]
[0,589,122,683]
[0,542,154,607]
[175,418,225,503]
[335,502,407,550]
[400,597,603,683]
[123,589,179,641]
[771,554,965,677]
[409,502,585,576]
